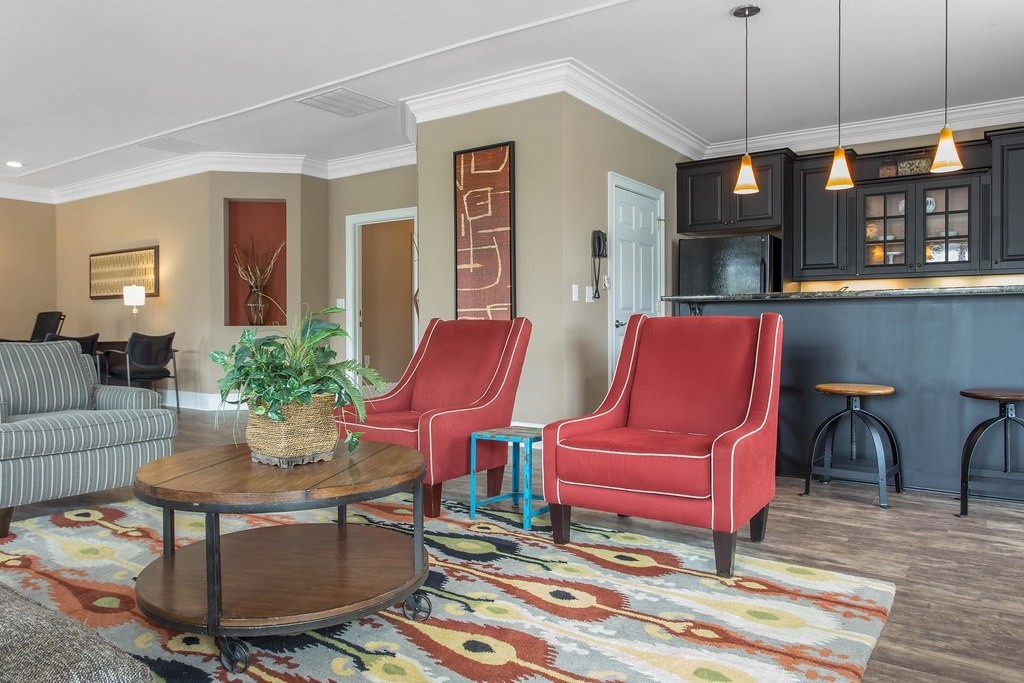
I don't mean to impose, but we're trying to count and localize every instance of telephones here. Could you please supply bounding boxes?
[592,230,608,258]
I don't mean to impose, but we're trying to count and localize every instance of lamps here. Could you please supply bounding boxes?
[123,284,146,332]
[732,4,761,195]
[825,0,856,190]
[929,0,963,175]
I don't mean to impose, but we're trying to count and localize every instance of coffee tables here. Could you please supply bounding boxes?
[134,437,434,673]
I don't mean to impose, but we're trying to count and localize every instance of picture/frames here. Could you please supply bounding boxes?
[88,244,160,300]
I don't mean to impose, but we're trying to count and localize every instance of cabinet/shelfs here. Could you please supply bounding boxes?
[677,146,789,234]
[990,130,1024,275]
[789,148,855,282]
[856,170,981,280]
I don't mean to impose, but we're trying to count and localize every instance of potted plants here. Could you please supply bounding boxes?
[208,303,389,470]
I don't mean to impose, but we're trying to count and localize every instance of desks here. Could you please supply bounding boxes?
[470,424,549,530]
[97,340,152,390]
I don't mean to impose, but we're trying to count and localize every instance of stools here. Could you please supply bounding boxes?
[953,387,1024,518]
[805,382,903,508]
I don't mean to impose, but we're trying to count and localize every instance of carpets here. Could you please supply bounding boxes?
[0,493,895,683]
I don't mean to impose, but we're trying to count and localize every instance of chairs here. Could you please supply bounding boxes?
[0,339,178,539]
[333,317,534,518]
[103,332,181,415]
[541,312,784,578]
[0,311,66,343]
[43,332,105,386]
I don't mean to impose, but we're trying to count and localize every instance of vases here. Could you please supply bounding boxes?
[244,285,269,326]
